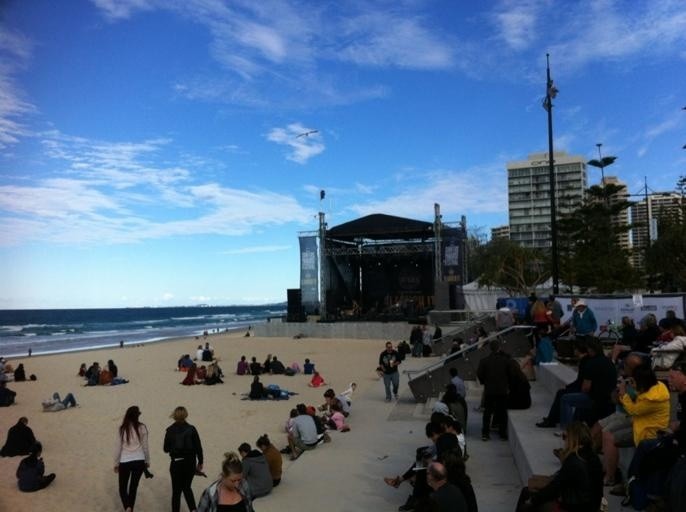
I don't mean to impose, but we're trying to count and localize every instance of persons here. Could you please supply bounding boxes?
[425,421,444,444]
[250,376,263,399]
[178,343,225,386]
[0,357,26,407]
[198,452,253,512]
[478,339,510,439]
[41,392,79,412]
[536,345,587,428]
[17,442,56,492]
[618,313,657,351]
[604,364,670,485]
[646,324,686,370]
[430,368,467,426]
[554,353,642,461]
[410,323,443,357]
[525,293,564,364]
[286,409,297,430]
[610,361,686,495]
[237,354,298,376]
[311,372,324,386]
[164,406,204,512]
[114,406,150,512]
[495,299,513,331]
[0,417,36,456]
[378,342,400,401]
[384,423,465,488]
[306,406,323,434]
[424,462,468,512]
[573,301,597,353]
[323,382,356,431]
[256,435,283,485]
[448,327,485,356]
[438,453,478,512]
[238,443,273,498]
[514,422,603,511]
[79,360,117,386]
[553,338,615,437]
[304,358,315,375]
[287,403,318,459]
[440,416,465,457]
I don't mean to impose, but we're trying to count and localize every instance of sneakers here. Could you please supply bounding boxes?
[382,476,403,490]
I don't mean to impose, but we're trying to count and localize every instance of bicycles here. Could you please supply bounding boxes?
[599,319,625,349]
